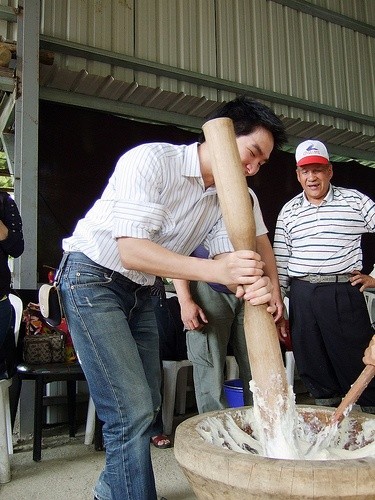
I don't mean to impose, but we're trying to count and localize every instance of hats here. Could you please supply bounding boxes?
[295,139,330,167]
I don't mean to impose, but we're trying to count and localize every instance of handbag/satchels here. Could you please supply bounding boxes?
[21,308,67,366]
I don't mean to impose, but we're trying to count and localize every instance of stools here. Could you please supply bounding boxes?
[84,355,241,445]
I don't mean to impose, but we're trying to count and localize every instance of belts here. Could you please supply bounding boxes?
[295,274,356,284]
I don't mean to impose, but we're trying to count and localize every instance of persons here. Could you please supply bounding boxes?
[149,276,172,450]
[173,185,281,414]
[0,189,24,433]
[273,139,375,415]
[363,335,375,368]
[54,99,287,500]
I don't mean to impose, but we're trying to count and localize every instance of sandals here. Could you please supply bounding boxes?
[150,433,172,449]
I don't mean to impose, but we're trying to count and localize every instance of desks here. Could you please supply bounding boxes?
[9,361,104,462]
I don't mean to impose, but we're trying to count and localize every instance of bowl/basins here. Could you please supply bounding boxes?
[222,379,244,407]
